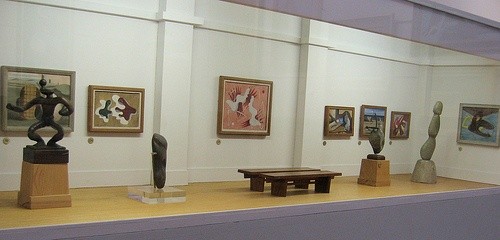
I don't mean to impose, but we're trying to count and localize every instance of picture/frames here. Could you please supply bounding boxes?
[457,103,500,147]
[0,66,76,131]
[390,111,411,138]
[324,106,355,136]
[359,105,387,138]
[88,85,145,133]
[217,76,273,136]
